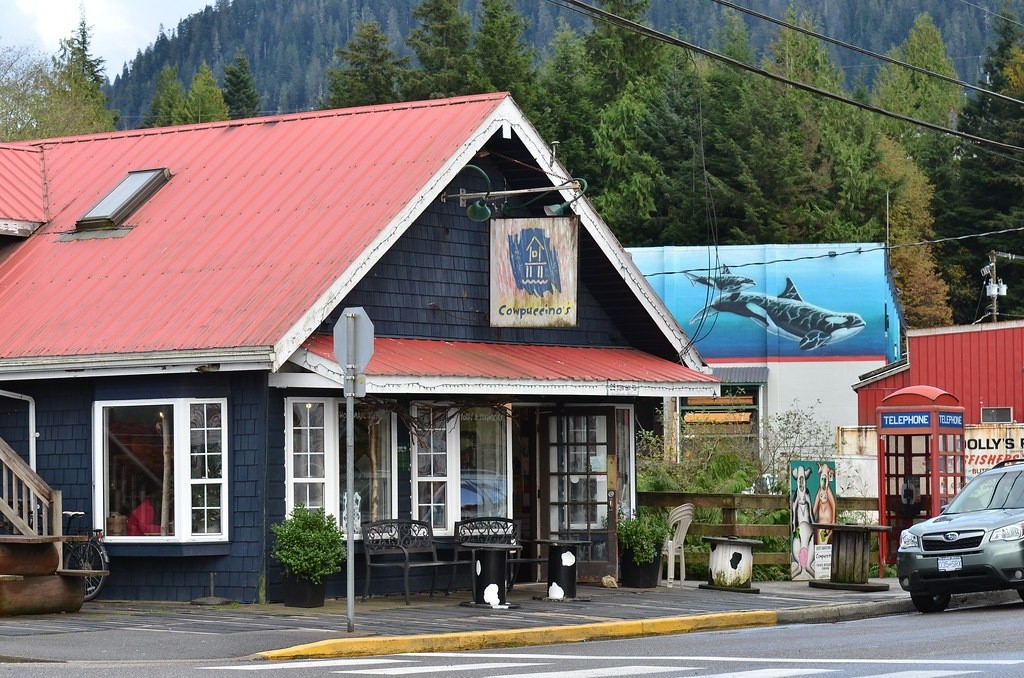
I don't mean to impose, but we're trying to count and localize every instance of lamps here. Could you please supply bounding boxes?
[458,164,588,221]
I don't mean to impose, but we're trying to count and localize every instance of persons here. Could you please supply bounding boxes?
[128,495,161,536]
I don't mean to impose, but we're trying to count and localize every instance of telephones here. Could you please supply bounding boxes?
[900,474,921,515]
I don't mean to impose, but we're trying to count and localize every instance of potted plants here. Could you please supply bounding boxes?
[270,500,346,608]
[603,510,673,589]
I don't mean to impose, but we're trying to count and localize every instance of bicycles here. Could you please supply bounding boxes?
[28,511,110,601]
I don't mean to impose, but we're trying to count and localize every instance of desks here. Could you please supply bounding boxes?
[533,539,595,602]
[0,535,89,616]
[459,543,523,609]
[698,535,764,594]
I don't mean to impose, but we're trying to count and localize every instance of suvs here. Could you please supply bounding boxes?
[895,458,1023,613]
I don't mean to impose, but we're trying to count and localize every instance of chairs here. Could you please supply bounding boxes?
[361,517,548,606]
[658,503,694,588]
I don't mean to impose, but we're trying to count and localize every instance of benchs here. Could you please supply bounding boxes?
[0,570,110,616]
